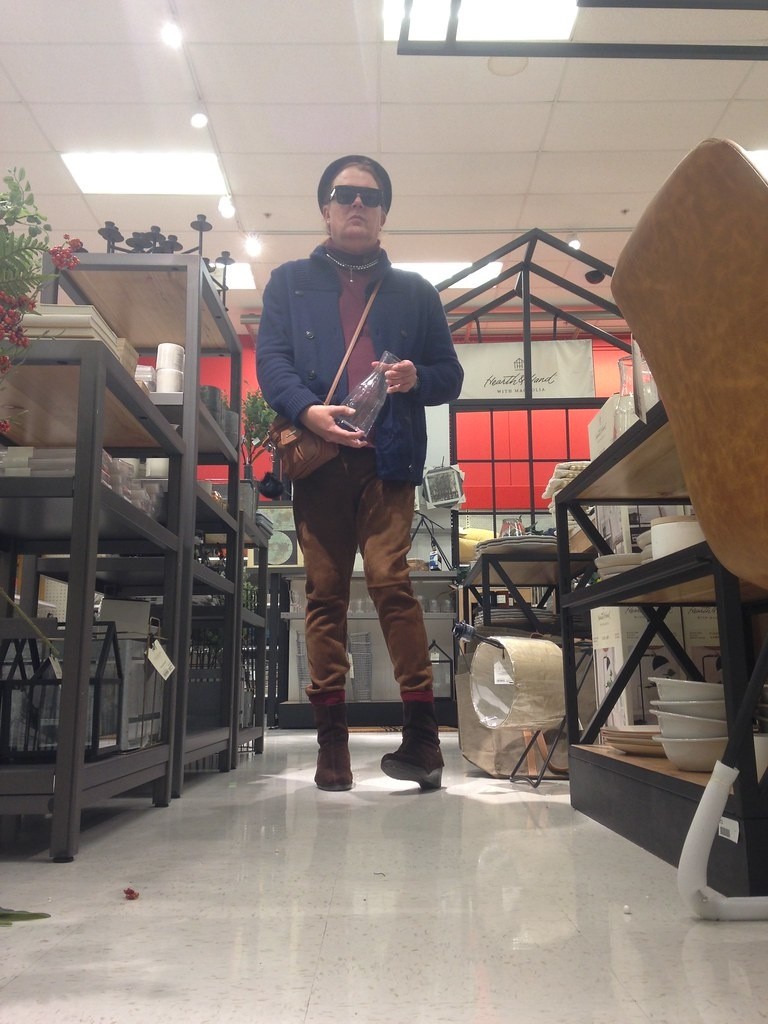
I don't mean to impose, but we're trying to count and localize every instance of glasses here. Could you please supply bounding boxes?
[327,185,384,207]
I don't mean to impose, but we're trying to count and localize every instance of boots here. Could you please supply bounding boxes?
[309,701,354,791]
[381,699,445,787]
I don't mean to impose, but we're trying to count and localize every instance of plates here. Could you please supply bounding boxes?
[594,529,653,581]
[601,725,666,758]
[475,535,558,554]
[258,532,294,564]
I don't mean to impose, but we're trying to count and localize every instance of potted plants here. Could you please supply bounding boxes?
[237,385,278,479]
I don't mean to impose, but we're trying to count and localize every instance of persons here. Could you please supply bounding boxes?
[252,154,466,793]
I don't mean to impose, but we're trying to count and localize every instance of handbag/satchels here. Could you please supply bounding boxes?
[262,412,340,481]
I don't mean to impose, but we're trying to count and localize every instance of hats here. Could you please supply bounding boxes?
[317,155,393,222]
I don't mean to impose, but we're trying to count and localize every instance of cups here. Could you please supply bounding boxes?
[155,343,185,393]
[295,632,373,702]
[291,590,456,614]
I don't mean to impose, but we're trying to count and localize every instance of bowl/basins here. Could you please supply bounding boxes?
[648,676,768,784]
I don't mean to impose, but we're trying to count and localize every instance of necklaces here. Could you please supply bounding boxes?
[325,251,380,283]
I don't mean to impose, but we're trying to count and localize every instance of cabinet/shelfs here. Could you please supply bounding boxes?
[0,253,768,900]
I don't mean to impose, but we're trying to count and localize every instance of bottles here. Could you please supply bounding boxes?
[613,356,661,441]
[336,351,402,437]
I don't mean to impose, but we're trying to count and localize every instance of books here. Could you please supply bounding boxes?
[117,338,156,397]
[0,304,121,362]
